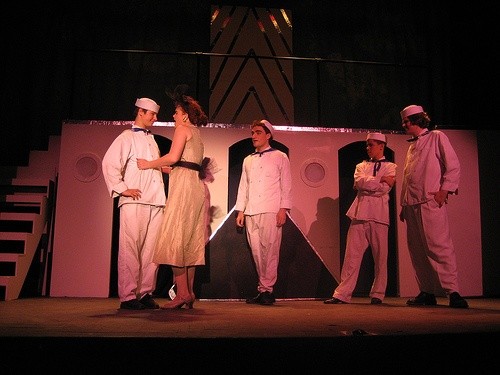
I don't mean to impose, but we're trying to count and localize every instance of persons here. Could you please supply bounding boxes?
[136,96,210,308]
[397,105,469,309]
[234,121,293,305]
[322,133,398,306]
[101,98,213,311]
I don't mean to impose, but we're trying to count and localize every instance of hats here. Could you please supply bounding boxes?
[366,133,388,143]
[260,120,274,140]
[135,98,160,114]
[400,105,424,118]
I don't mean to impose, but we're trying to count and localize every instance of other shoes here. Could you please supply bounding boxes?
[120,299,149,313]
[246,292,275,306]
[407,294,438,307]
[139,293,160,311]
[450,293,469,308]
[371,297,383,305]
[324,297,343,304]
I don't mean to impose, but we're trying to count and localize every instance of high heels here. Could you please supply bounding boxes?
[159,294,196,309]
